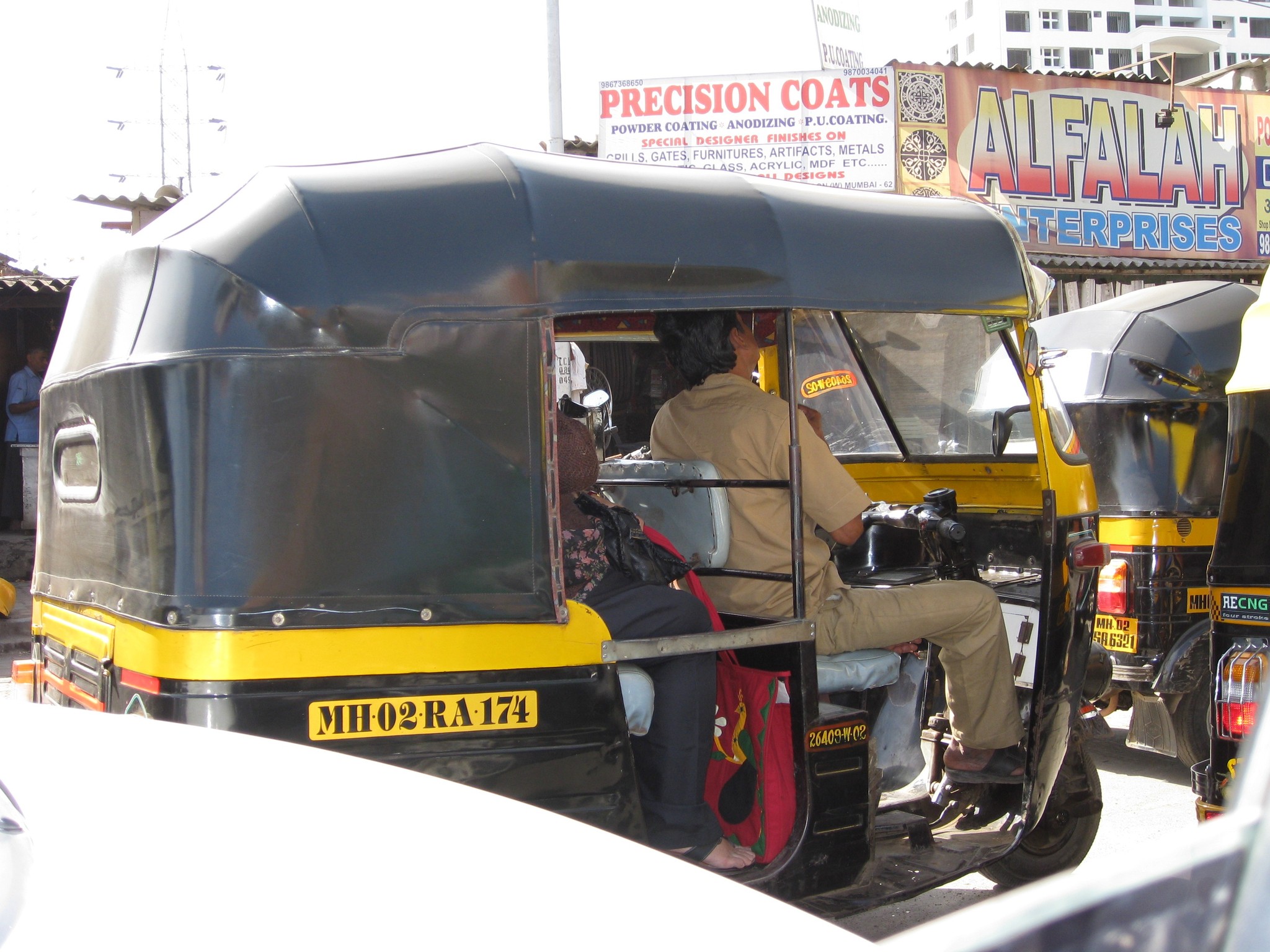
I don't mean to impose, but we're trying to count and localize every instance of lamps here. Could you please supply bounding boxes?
[1094,52,1178,129]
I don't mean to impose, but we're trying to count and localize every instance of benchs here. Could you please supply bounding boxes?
[558,458,903,736]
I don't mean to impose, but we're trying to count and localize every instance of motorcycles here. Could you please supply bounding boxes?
[1190,258,1270,825]
[966,280,1262,770]
[9,144,1114,917]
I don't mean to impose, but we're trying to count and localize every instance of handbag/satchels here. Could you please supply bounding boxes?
[578,496,694,588]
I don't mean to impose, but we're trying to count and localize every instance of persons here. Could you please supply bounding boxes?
[556,401,756,876]
[650,308,1028,784]
[4,344,49,531]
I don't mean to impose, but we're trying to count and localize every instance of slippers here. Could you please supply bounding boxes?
[664,837,754,879]
[946,745,1030,784]
